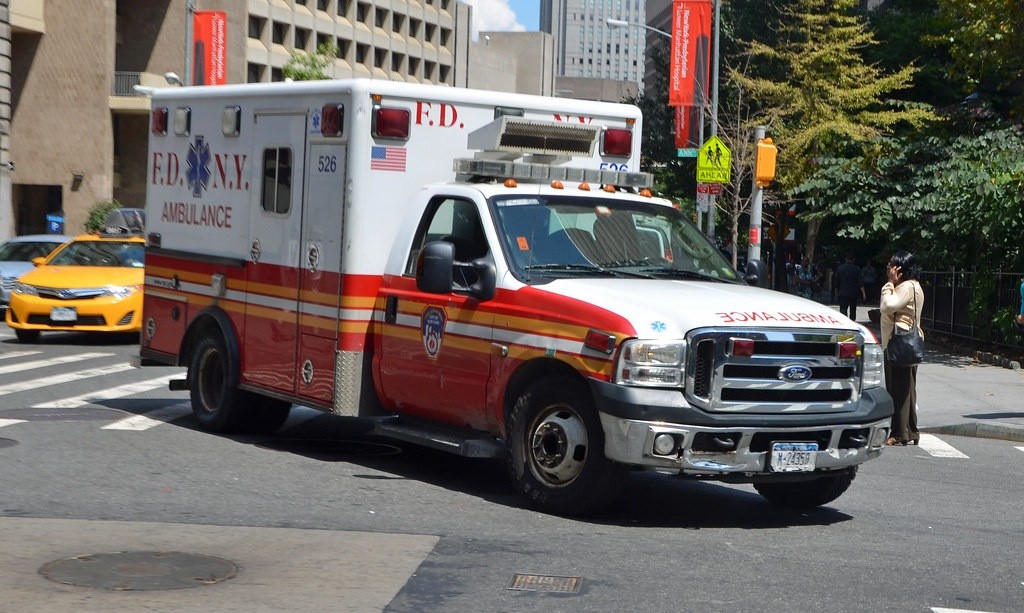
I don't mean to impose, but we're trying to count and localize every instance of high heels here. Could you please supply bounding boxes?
[908,439,919,445]
[886,436,908,446]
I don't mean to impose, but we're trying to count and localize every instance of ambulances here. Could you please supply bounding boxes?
[129,78,894,506]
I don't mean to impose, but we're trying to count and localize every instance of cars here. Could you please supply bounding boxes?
[5,208,146,345]
[0,234,72,322]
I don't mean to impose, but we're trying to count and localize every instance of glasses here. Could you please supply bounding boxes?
[887,260,895,266]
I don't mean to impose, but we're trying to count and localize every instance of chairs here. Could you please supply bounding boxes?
[538,227,599,268]
[439,234,487,286]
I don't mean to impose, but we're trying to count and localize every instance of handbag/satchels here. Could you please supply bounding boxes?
[886,317,925,367]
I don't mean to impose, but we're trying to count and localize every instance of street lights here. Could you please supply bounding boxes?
[606,19,705,233]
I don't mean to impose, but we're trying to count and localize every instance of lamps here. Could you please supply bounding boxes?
[71,171,86,180]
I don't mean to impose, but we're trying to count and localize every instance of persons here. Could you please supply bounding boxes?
[833,250,865,322]
[786,256,824,300]
[861,259,877,304]
[569,213,640,265]
[1017,277,1024,324]
[880,250,924,445]
